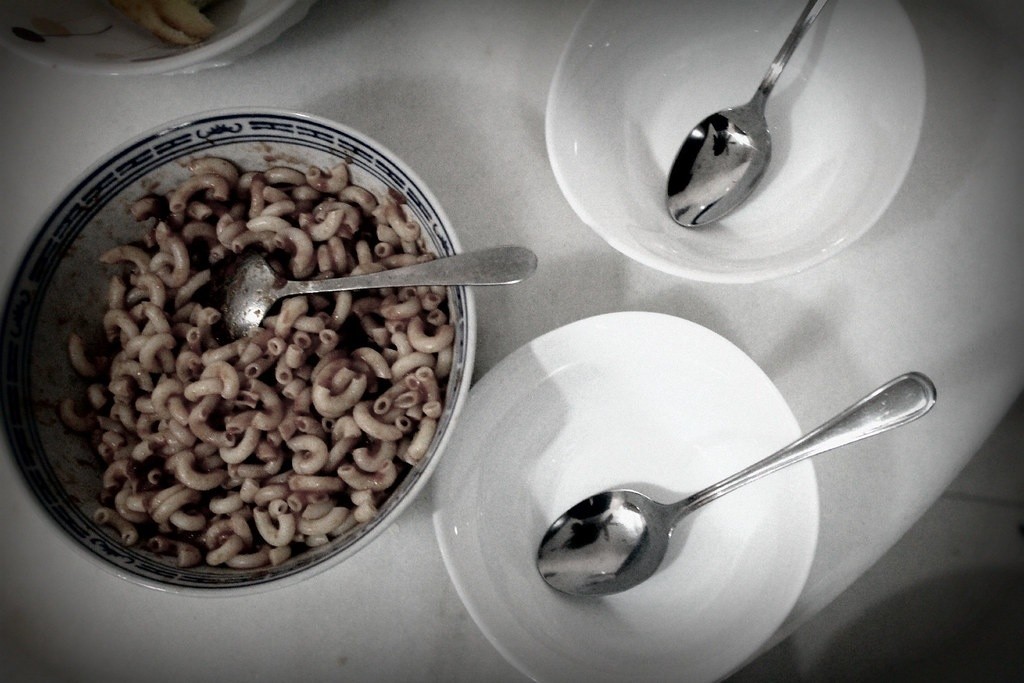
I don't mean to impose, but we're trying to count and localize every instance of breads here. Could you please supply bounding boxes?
[106,0,217,46]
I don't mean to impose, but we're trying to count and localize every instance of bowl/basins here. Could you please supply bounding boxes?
[0,1,315,86]
[544,0,926,286]
[433,310,821,683]
[0,105,479,599]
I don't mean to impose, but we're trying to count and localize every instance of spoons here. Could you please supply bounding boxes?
[217,247,537,338]
[665,0,835,228]
[536,372,936,595]
